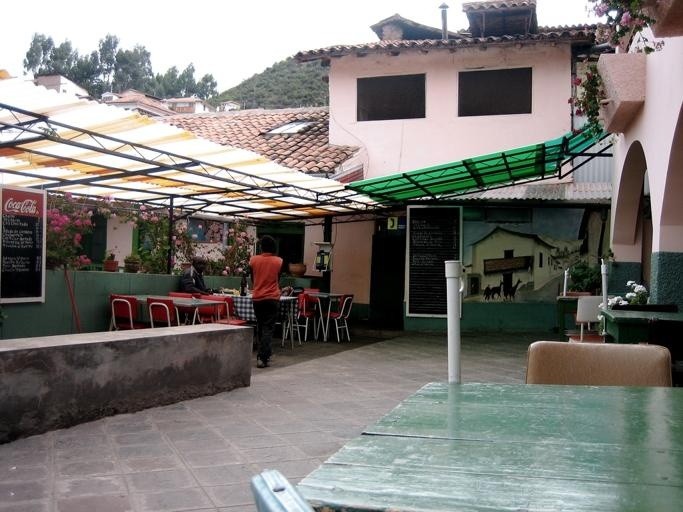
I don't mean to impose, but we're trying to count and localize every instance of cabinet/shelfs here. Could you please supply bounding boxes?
[239,273,248,297]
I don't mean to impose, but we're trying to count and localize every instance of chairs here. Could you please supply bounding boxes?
[526,291,682,387]
[108,287,354,353]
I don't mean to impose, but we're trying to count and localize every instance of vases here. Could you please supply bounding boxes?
[47,192,258,276]
[568,0,664,145]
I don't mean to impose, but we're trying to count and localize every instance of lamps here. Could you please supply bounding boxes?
[108,287,354,353]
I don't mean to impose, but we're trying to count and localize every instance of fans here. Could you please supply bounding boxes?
[0,184,47,303]
[406,205,463,319]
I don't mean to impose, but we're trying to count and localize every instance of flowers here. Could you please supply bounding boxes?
[568,0,664,145]
[47,192,258,276]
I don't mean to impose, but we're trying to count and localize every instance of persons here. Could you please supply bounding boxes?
[248,234,283,368]
[179,255,214,296]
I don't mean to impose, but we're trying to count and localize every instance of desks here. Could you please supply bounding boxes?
[295,381,683,512]
